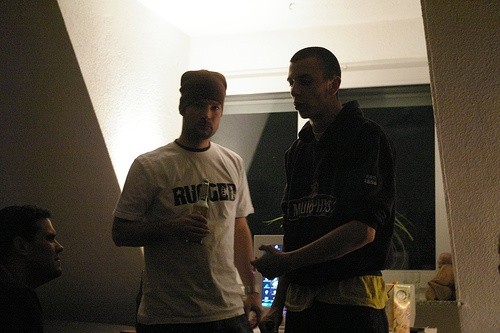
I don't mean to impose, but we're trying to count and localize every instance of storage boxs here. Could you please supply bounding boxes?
[386,282,416,333]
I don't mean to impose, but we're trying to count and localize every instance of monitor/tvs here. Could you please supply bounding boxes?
[253,234,284,312]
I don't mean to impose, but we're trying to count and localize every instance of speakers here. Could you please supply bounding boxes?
[384,284,416,327]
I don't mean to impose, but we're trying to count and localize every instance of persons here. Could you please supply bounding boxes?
[250,47,397,333]
[0,206,64,333]
[111,70,266,333]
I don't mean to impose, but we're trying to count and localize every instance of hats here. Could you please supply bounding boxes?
[178,70,227,114]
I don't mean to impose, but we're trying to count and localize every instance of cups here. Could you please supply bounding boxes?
[405,272,421,299]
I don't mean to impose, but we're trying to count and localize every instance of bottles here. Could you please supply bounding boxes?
[186,177,209,245]
